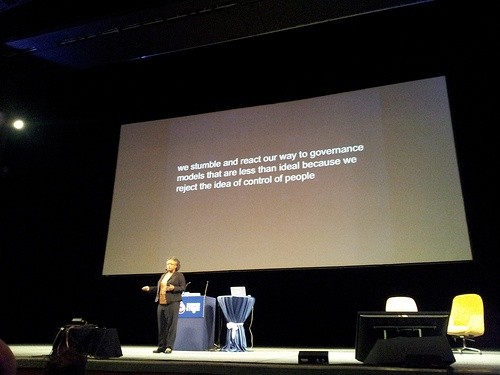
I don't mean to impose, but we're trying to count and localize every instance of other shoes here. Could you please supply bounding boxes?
[165,351,171,353]
[153,349,164,353]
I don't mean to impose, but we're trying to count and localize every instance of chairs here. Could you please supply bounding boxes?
[383,296,422,340]
[446,293,485,356]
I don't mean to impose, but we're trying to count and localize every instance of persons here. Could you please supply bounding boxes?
[0,338,88,375]
[142,256,186,353]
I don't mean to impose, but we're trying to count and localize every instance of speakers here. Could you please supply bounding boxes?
[364,336,456,368]
[55,328,123,358]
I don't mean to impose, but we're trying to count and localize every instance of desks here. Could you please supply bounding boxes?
[217,295,255,352]
[355,311,452,363]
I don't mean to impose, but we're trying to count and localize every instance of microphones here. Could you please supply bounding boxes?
[203,281,208,296]
[185,282,191,286]
